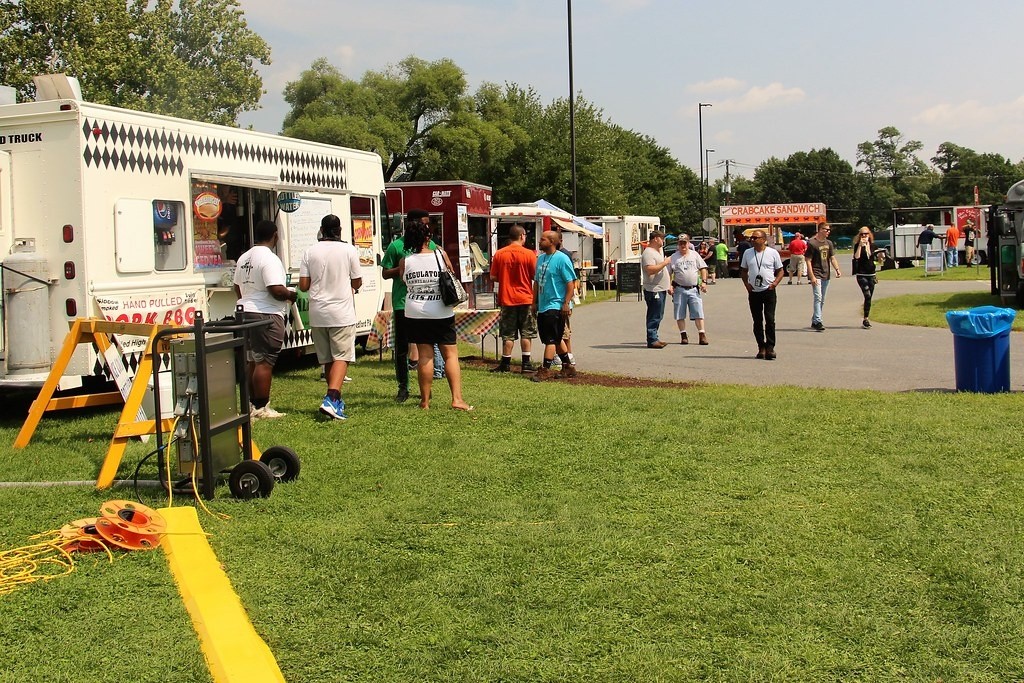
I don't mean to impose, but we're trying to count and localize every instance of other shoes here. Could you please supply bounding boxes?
[648,331,777,361]
[708,281,716,285]
[320,372,352,383]
[811,322,825,330]
[788,280,812,285]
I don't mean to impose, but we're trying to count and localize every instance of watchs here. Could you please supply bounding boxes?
[702,279,707,283]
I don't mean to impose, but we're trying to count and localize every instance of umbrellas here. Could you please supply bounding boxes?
[743,227,796,245]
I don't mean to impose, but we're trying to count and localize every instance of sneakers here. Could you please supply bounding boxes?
[319,397,347,420]
[394,352,577,410]
[249,401,287,419]
[863,318,872,328]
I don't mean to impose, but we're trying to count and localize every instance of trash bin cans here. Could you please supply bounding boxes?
[946,304,1016,392]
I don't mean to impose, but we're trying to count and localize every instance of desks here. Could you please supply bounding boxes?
[365,305,502,364]
[579,266,598,298]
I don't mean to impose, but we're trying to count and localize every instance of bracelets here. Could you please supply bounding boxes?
[836,269,838,271]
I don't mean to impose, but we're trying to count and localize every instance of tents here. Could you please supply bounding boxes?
[533,199,612,294]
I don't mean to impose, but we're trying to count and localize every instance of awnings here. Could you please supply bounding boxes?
[468,205,572,248]
[551,216,603,247]
[197,170,352,225]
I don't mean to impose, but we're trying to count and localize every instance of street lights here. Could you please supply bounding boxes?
[699,102,712,242]
[706,150,715,218]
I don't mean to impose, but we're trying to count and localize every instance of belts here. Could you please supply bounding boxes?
[677,284,697,290]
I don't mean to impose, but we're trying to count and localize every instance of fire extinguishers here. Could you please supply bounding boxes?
[609,260,615,275]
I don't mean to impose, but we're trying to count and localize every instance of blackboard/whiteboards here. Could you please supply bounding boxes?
[616,261,642,293]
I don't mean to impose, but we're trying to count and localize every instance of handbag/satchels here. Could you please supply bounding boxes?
[433,246,468,307]
[852,259,859,275]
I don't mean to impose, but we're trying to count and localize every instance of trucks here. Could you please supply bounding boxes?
[0,75,387,393]
[575,215,661,283]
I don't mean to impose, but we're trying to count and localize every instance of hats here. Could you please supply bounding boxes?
[928,224,934,229]
[322,215,340,228]
[795,232,802,237]
[678,233,690,242]
[651,230,667,237]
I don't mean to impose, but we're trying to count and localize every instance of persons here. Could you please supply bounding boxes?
[804,222,841,332]
[737,235,750,267]
[963,220,981,268]
[488,226,539,373]
[666,233,709,345]
[716,239,729,272]
[852,227,885,329]
[230,219,298,420]
[787,232,812,285]
[696,242,709,283]
[642,230,671,346]
[702,237,717,286]
[944,221,959,268]
[916,224,943,269]
[530,229,578,382]
[298,213,362,420]
[740,230,784,360]
[380,209,471,411]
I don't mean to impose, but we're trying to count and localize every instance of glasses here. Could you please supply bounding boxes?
[860,233,869,236]
[750,236,763,240]
[822,228,830,232]
[701,243,706,245]
[421,222,430,229]
[521,234,527,237]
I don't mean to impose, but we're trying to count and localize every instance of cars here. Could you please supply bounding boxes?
[663,235,718,257]
